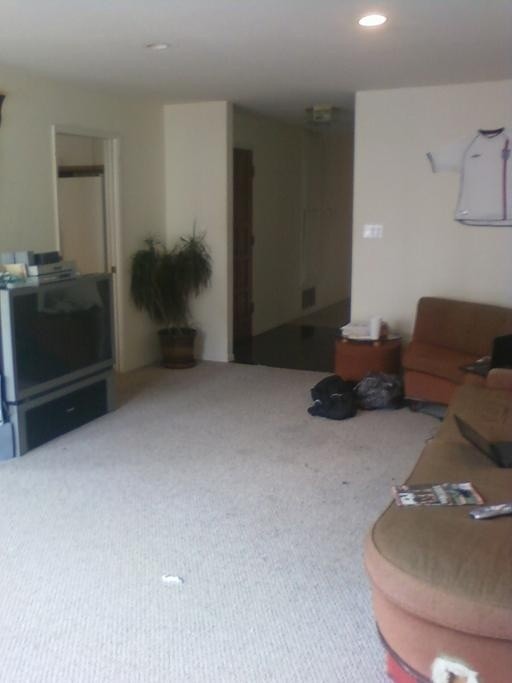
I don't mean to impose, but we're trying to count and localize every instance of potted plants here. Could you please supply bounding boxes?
[131,224,211,369]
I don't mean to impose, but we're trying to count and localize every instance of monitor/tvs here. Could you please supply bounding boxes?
[0,271,117,402]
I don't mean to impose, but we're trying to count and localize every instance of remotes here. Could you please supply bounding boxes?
[470,503,512,520]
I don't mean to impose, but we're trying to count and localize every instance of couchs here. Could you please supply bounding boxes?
[366,294,512,683]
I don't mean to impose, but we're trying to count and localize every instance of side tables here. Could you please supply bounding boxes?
[335,332,404,382]
